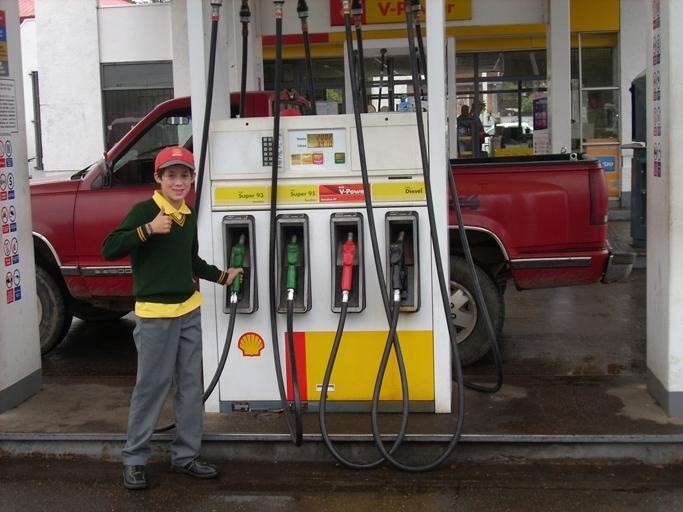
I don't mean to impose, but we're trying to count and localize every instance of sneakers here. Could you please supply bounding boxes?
[170,458,217,480]
[121,462,149,490]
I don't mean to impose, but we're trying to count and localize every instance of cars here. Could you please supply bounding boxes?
[485,120,533,138]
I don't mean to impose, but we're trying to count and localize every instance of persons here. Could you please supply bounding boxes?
[464,101,486,152]
[456,122,471,153]
[101,145,246,492]
[455,103,470,126]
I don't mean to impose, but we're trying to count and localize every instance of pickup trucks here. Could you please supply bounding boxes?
[30,90,613,367]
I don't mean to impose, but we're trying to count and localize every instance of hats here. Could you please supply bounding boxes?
[154,146,195,172]
